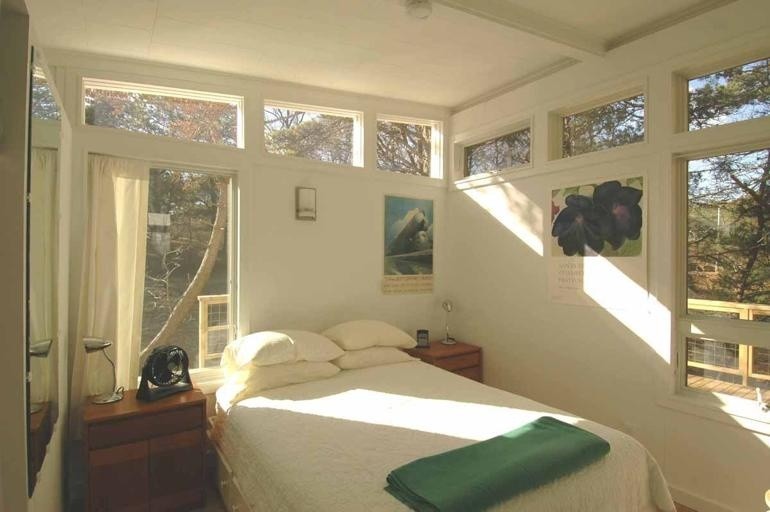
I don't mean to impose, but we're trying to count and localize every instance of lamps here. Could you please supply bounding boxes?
[30,340,53,413]
[404,1,435,22]
[82,336,123,405]
[439,299,459,346]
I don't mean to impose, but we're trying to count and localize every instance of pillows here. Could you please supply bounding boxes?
[221,329,345,362]
[324,318,417,351]
[215,363,345,389]
[334,347,419,370]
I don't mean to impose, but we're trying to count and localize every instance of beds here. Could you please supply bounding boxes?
[211,356,676,512]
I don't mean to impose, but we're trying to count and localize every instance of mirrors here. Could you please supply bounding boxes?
[24,48,60,498]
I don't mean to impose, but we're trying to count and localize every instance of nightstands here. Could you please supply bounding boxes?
[30,399,52,492]
[407,330,482,384]
[85,385,207,512]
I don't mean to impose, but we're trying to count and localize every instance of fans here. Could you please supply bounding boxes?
[136,345,197,402]
